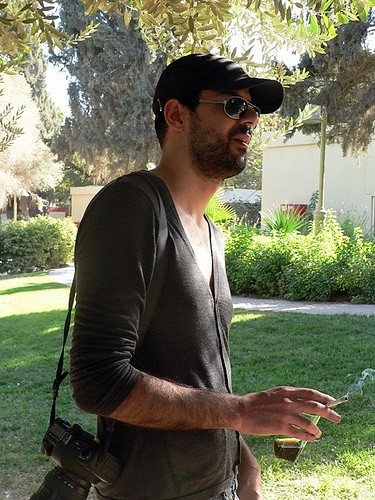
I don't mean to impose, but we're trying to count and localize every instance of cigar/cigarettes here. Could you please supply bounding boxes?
[325,394,350,413]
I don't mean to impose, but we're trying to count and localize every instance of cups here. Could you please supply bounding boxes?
[273,410,320,463]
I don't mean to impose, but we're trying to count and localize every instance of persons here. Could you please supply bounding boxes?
[80,53,343,499]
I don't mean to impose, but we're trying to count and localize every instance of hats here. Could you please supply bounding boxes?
[152,52,284,114]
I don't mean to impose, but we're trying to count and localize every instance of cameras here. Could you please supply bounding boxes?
[28,417,122,500]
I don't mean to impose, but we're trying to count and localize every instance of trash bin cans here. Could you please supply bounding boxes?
[280,202,308,231]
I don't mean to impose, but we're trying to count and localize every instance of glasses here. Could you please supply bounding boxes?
[198,94,261,122]
